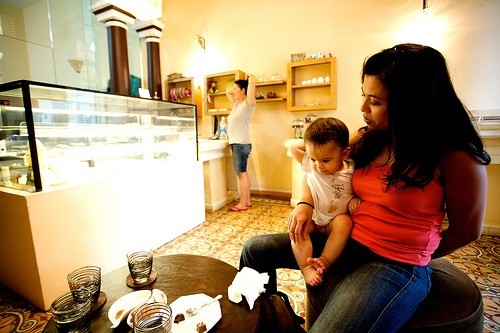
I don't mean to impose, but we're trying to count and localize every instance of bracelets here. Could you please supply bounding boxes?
[297,202,314,209]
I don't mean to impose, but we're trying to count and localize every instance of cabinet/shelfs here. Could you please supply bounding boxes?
[163,57,337,118]
[0,80,206,311]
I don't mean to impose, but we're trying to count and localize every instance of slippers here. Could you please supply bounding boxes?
[229,200,252,212]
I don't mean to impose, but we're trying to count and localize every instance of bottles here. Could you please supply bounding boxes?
[219,117,227,141]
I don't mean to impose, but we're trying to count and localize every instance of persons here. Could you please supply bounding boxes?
[226,74,258,211]
[240,43,491,333]
[288,117,362,285]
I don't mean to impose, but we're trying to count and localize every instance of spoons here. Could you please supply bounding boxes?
[185,294,223,315]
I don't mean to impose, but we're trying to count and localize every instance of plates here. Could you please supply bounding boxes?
[161,293,222,333]
[108,289,167,325]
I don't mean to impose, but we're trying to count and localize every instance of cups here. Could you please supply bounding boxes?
[50,288,93,333]
[133,303,172,333]
[67,265,101,305]
[267,92,275,99]
[126,249,153,284]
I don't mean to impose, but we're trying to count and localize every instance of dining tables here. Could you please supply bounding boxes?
[40,255,262,333]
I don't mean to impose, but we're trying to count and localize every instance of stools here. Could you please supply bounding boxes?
[305,258,483,333]
